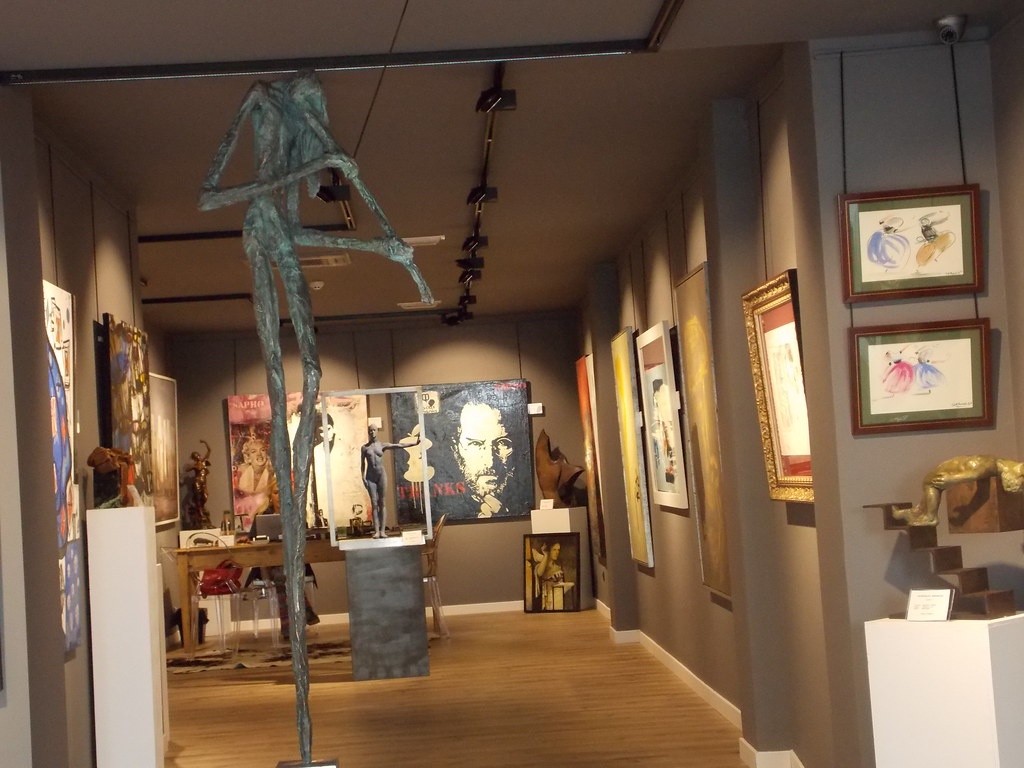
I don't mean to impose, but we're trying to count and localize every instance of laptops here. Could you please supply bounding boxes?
[256,514,283,541]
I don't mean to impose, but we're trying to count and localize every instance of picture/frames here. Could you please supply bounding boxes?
[836,184,984,305]
[674,263,733,601]
[145,372,180,526]
[849,318,992,436]
[522,533,581,612]
[741,267,814,506]
[611,326,656,568]
[637,322,688,510]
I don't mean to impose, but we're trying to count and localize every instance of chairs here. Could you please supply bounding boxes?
[422,514,447,633]
[161,546,314,657]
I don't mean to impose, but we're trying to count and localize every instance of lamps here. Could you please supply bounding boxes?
[477,89,517,113]
[461,236,489,250]
[459,295,477,304]
[458,271,481,282]
[455,257,485,271]
[317,183,351,204]
[467,187,498,206]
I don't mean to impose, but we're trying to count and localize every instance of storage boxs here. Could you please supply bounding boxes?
[943,478,1024,534]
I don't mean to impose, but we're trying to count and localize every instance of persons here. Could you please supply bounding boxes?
[244,480,321,645]
[536,540,562,578]
[182,438,217,530]
[318,413,335,442]
[360,424,422,539]
[450,383,517,519]
[236,438,277,511]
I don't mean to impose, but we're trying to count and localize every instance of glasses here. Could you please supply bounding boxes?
[458,438,513,458]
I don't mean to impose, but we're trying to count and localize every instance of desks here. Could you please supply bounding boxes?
[531,508,594,610]
[339,535,429,681]
[170,539,344,651]
[179,529,233,636]
[86,505,164,768]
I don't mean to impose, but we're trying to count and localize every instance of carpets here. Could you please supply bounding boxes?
[166,633,451,674]
